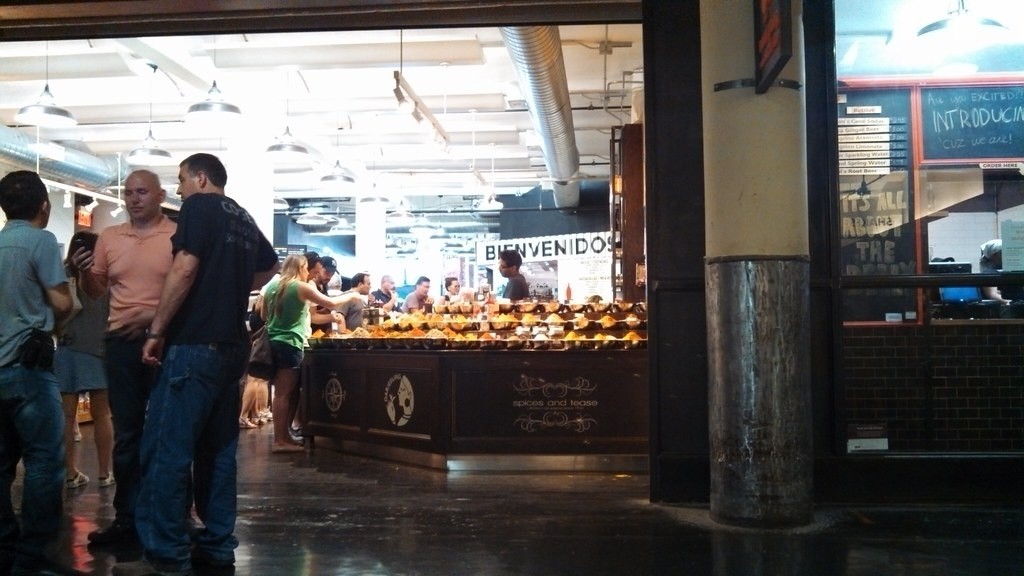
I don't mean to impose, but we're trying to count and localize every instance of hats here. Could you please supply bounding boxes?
[319,256,339,274]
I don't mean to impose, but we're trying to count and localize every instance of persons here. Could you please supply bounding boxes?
[260,254,361,454]
[135,153,281,576]
[586,295,603,304]
[71,170,177,562]
[498,250,530,303]
[0,170,84,576]
[51,231,114,491]
[927,238,1012,305]
[239,251,473,427]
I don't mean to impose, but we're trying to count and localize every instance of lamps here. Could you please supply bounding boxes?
[14,41,77,128]
[265,97,309,158]
[319,133,355,187]
[110,204,124,217]
[63,188,72,209]
[392,78,410,112]
[125,92,173,165]
[411,101,430,130]
[187,35,244,120]
[917,0,1010,49]
[273,193,504,256]
[80,198,99,216]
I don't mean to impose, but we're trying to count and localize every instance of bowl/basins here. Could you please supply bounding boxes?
[369,303,647,348]
[307,338,367,348]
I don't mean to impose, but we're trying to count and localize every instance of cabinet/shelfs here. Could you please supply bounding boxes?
[609,122,643,304]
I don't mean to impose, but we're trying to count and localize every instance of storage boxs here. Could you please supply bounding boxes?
[364,309,384,325]
[846,438,889,452]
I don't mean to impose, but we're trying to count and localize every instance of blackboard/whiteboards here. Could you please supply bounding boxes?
[914,77,1024,166]
[832,76,926,331]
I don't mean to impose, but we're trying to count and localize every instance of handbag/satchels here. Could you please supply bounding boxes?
[50,276,83,334]
[248,326,273,379]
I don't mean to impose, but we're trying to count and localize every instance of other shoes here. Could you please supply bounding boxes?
[111,558,193,576]
[88,519,138,544]
[189,544,234,567]
[288,432,303,444]
[185,518,240,548]
[269,445,305,454]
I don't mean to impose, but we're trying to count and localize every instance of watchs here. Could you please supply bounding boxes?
[145,326,163,339]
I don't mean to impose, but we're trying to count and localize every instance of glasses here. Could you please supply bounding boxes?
[497,261,512,269]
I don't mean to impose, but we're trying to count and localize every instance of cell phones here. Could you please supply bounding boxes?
[75,239,95,266]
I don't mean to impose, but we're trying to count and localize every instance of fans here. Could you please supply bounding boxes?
[840,175,885,196]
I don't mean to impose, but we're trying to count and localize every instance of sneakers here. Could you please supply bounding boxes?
[98,473,115,488]
[65,471,91,489]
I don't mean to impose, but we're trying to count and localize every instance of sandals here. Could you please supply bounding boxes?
[235,408,274,429]
